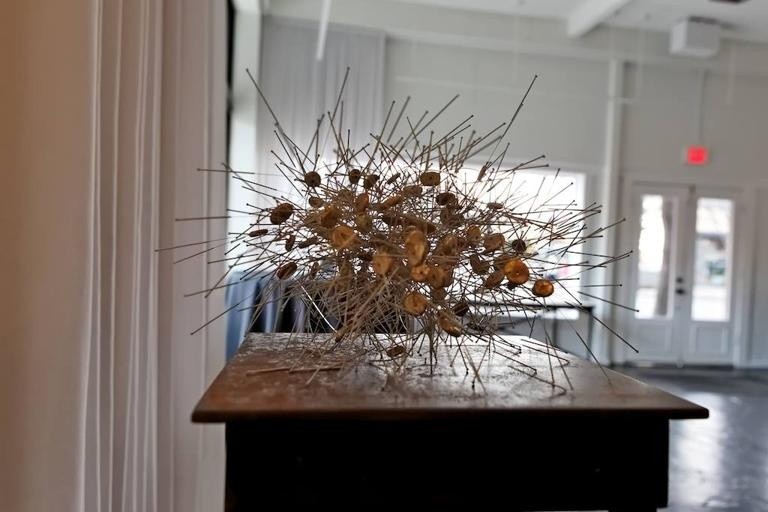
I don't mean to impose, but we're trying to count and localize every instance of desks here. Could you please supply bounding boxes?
[223,273,411,356]
[190,336,709,512]
[437,298,594,361]
